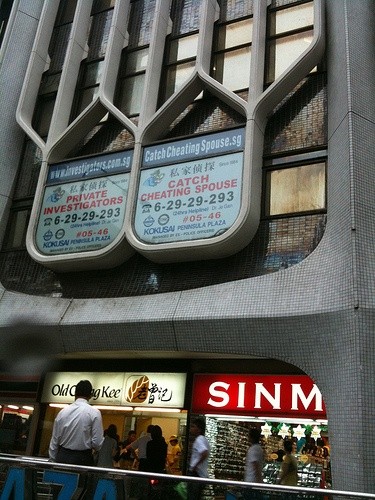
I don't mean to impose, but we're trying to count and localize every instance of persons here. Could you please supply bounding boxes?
[104,423,181,475]
[239,426,329,485]
[49,380,105,466]
[186,418,209,500]
